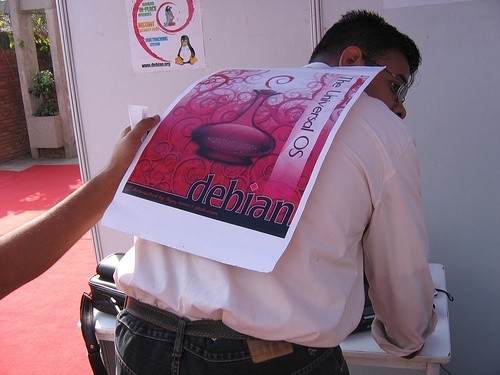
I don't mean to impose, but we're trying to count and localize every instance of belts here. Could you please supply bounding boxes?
[123,296,250,340]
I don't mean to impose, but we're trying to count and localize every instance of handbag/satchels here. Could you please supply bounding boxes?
[88,253,130,315]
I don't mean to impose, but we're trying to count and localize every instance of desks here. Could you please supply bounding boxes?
[77,262,452,375]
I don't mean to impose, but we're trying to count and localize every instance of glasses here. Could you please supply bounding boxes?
[363,55,409,103]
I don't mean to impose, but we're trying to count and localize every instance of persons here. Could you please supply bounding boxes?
[113,12,437,375]
[0,113,161,295]
